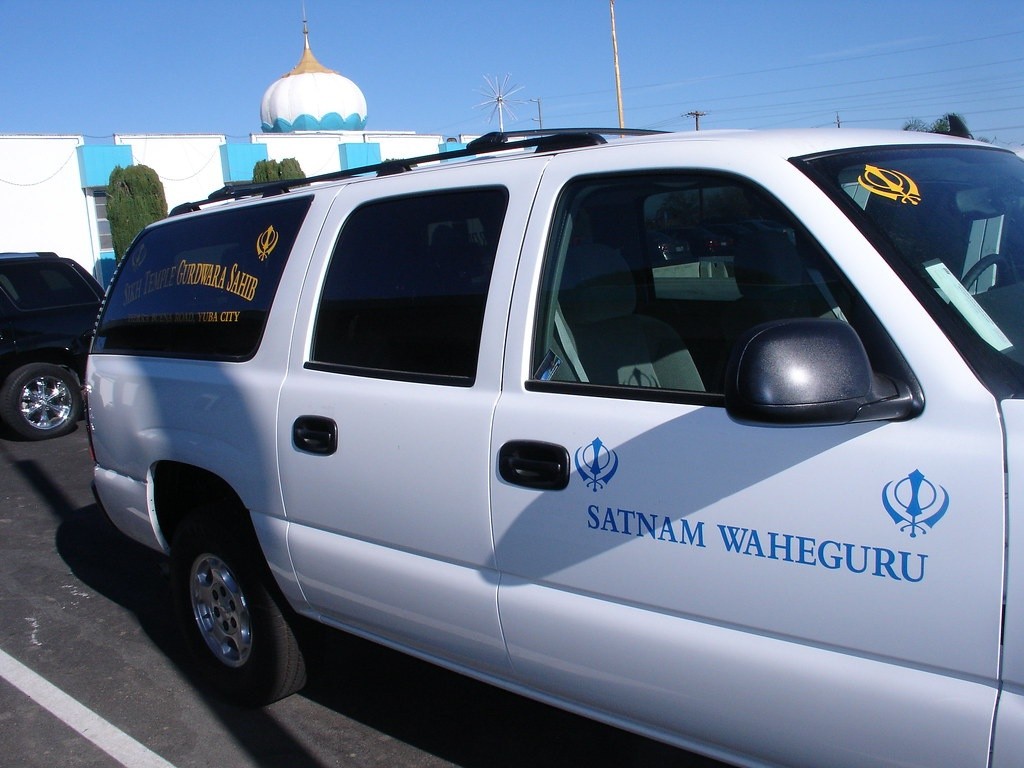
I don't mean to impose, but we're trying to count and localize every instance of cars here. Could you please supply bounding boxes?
[0,251,107,441]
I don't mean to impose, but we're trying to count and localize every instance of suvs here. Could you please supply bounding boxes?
[84,127,1024,768]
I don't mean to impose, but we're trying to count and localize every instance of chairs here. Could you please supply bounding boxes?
[552,214,846,390]
